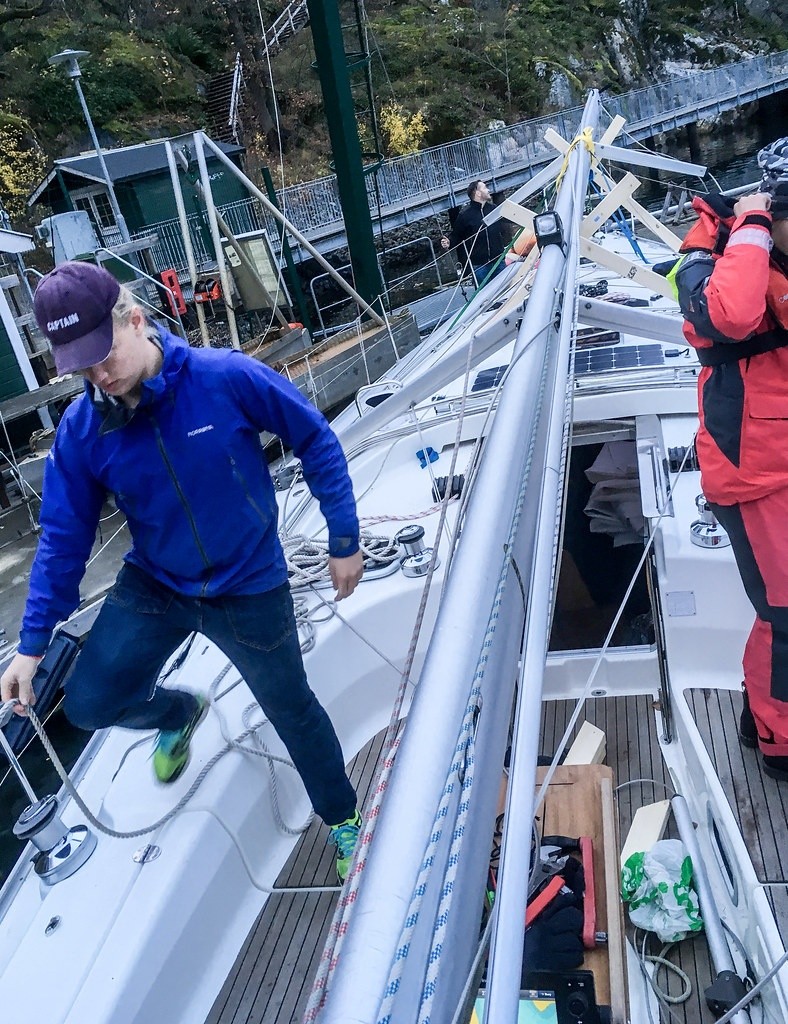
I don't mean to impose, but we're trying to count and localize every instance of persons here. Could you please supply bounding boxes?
[441,180,513,288]
[0,261,367,887]
[666,139,788,785]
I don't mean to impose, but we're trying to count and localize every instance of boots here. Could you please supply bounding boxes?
[763,753,788,781]
[739,689,759,747]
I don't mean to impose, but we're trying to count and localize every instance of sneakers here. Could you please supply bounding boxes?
[149,696,210,783]
[328,809,362,886]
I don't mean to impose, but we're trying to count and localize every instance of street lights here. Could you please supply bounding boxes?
[47,48,153,310]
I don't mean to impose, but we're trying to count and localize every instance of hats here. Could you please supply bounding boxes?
[34,262,119,376]
[756,136,788,218]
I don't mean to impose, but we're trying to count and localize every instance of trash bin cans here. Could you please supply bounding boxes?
[71,250,149,305]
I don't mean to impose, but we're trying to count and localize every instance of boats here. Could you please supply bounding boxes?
[1,125,531,753]
